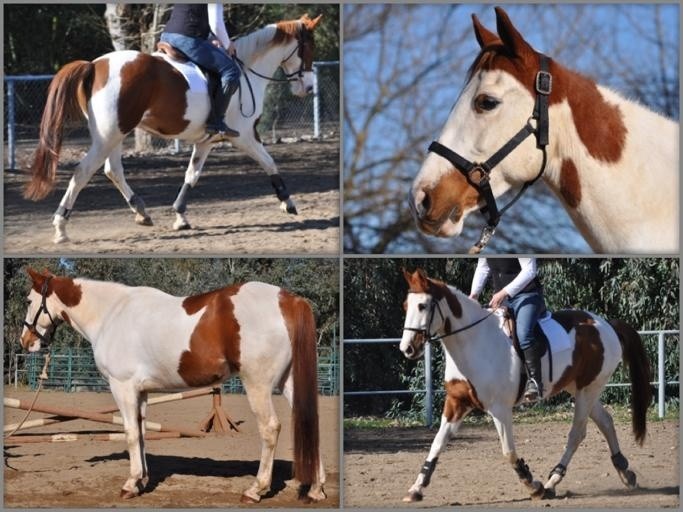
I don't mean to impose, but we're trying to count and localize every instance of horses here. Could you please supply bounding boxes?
[18,12,325,245]
[19,266,328,505]
[398,266,653,504]
[405,4,681,257]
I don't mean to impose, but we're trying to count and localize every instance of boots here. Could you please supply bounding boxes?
[205,84,240,137]
[524,349,542,401]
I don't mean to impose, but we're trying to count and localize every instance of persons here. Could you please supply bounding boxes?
[467,258,547,401]
[159,4,241,137]
